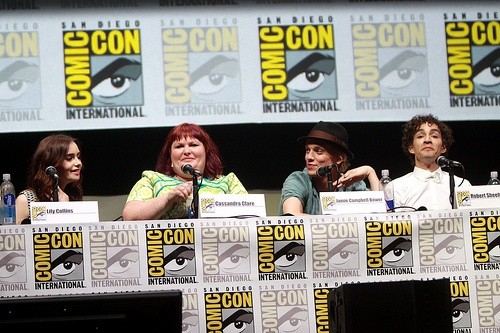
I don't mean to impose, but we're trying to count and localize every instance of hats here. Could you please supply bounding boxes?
[297,121,354,160]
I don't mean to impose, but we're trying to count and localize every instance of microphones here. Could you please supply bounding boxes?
[45,165,59,180]
[435,155,462,167]
[316,162,337,178]
[180,163,200,176]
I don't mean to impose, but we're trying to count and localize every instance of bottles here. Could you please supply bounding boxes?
[0,174,16,225]
[487,171,500,185]
[379,169,394,212]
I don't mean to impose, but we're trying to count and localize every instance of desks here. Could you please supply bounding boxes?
[0,209,500,332]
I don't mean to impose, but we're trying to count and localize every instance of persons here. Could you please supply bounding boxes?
[380,114,473,211]
[13,135,84,227]
[122,123,250,221]
[275,122,383,216]
